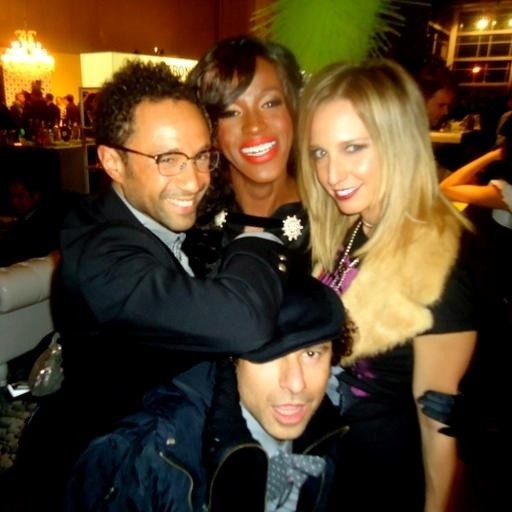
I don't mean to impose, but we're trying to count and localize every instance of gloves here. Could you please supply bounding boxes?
[420,390,480,439]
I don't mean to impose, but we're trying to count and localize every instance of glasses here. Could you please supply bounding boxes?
[106,145,220,177]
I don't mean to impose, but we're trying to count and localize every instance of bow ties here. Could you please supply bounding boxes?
[265,451,327,503]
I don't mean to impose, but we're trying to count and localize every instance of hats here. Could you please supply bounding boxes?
[235,272,345,363]
